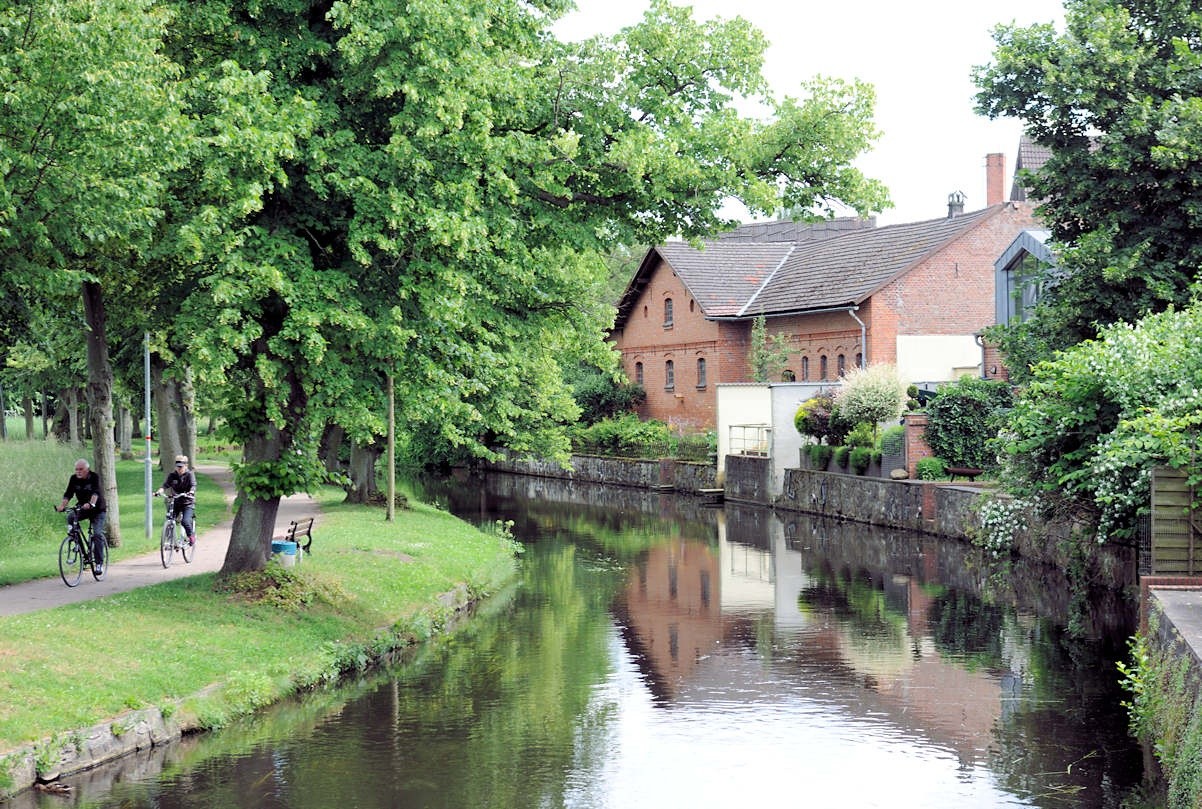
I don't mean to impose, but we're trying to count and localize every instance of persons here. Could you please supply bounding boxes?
[58,458,107,576]
[154,455,197,545]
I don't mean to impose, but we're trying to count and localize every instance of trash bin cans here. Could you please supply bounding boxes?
[272,542,297,567]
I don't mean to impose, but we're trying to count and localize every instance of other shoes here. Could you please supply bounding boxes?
[189,536,195,546]
[96,564,102,575]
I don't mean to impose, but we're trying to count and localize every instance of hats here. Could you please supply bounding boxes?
[175,455,188,467]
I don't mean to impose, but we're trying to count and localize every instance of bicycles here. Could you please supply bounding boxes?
[151,491,196,569]
[54,504,108,587]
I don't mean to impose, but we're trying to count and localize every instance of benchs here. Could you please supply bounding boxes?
[272,517,314,556]
